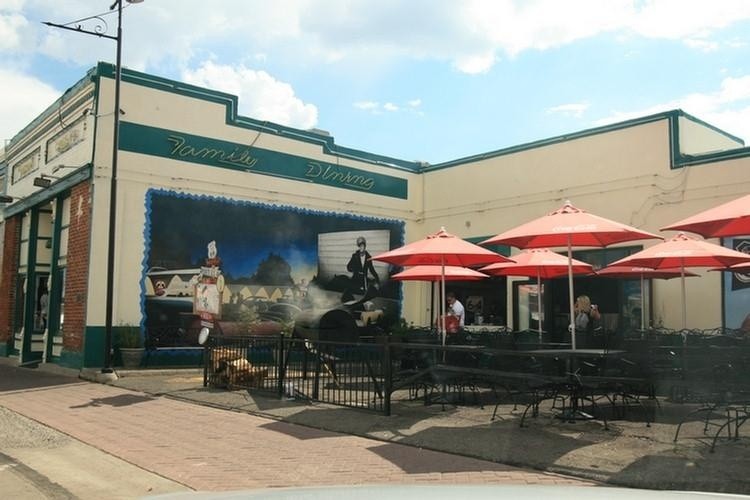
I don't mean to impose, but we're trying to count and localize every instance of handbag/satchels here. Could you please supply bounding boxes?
[575,313,589,332]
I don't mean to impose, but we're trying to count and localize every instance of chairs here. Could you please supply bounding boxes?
[388,328,750,452]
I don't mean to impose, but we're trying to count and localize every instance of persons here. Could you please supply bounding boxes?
[346,235,381,304]
[446,293,465,330]
[575,296,603,332]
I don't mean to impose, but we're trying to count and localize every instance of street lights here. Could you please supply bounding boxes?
[42,0,145,373]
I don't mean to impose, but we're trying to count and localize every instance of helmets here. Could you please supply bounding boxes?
[357,237,366,253]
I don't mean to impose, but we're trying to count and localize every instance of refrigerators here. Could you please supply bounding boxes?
[512,281,559,329]
